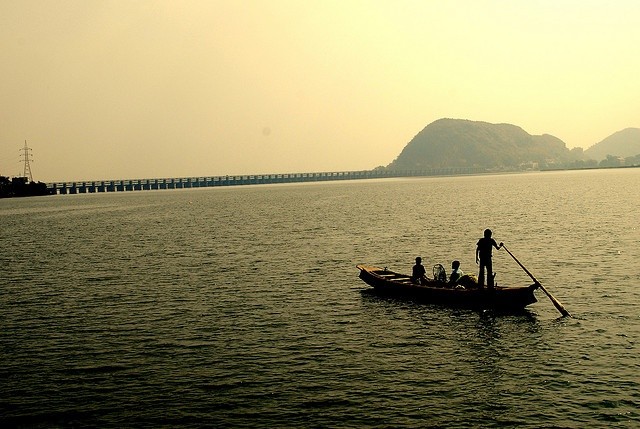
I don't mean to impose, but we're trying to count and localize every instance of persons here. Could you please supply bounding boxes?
[412,257,427,285]
[447,261,464,289]
[476,229,503,288]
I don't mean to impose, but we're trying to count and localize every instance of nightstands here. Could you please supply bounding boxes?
[502,245,574,318]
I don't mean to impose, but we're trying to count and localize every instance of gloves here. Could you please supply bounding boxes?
[356,263,541,311]
